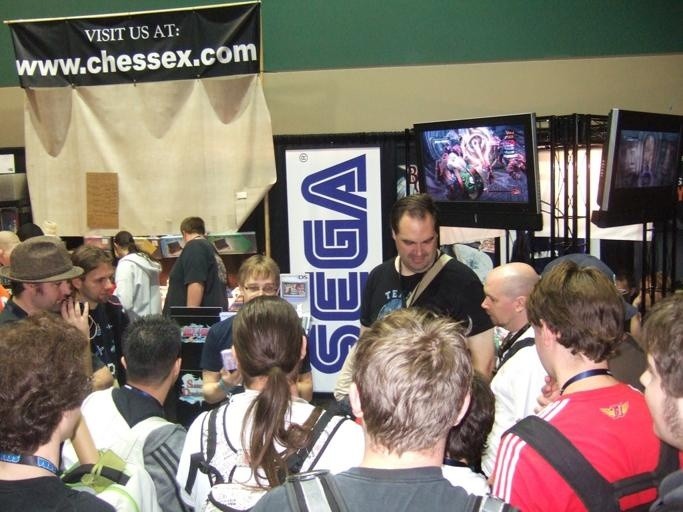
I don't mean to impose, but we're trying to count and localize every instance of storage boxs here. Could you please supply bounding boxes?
[61,232,264,259]
[0,172,29,202]
[0,146,26,173]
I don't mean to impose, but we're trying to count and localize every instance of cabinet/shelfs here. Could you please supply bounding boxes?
[0,199,32,234]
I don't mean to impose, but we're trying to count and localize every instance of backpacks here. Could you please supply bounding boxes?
[184,402,350,512]
[59,415,176,512]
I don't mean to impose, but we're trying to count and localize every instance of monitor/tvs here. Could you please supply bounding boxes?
[411,113,544,229]
[591,108,683,227]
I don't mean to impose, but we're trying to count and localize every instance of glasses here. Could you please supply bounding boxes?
[243,285,278,294]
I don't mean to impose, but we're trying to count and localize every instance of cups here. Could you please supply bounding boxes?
[219,349,238,372]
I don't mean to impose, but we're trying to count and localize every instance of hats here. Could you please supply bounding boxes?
[0,235,85,283]
[539,253,639,322]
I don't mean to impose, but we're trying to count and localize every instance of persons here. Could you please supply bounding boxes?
[16,222,44,244]
[357,194,496,383]
[0,230,24,266]
[1,236,683,511]
[161,216,228,319]
[111,230,163,321]
